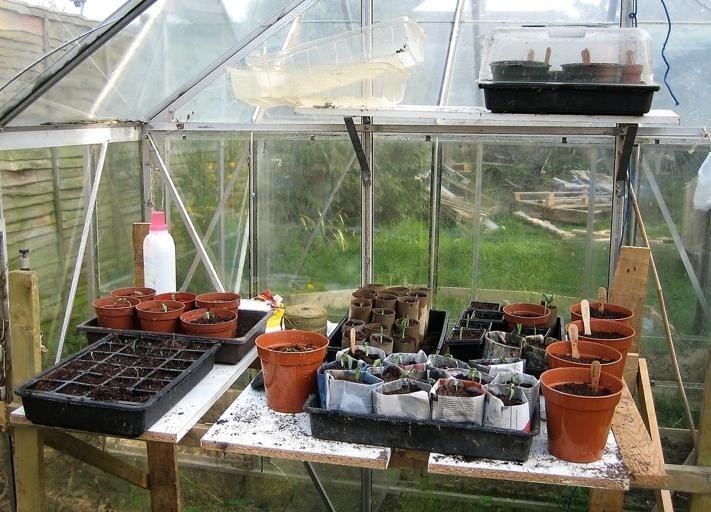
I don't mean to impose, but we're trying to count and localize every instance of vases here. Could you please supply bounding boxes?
[539,298,636,464]
[490,59,645,85]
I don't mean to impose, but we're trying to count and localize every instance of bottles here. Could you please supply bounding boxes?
[142,211,177,296]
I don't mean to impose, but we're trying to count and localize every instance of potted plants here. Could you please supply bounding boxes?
[14,287,243,438]
[255,328,329,413]
[316,282,560,432]
